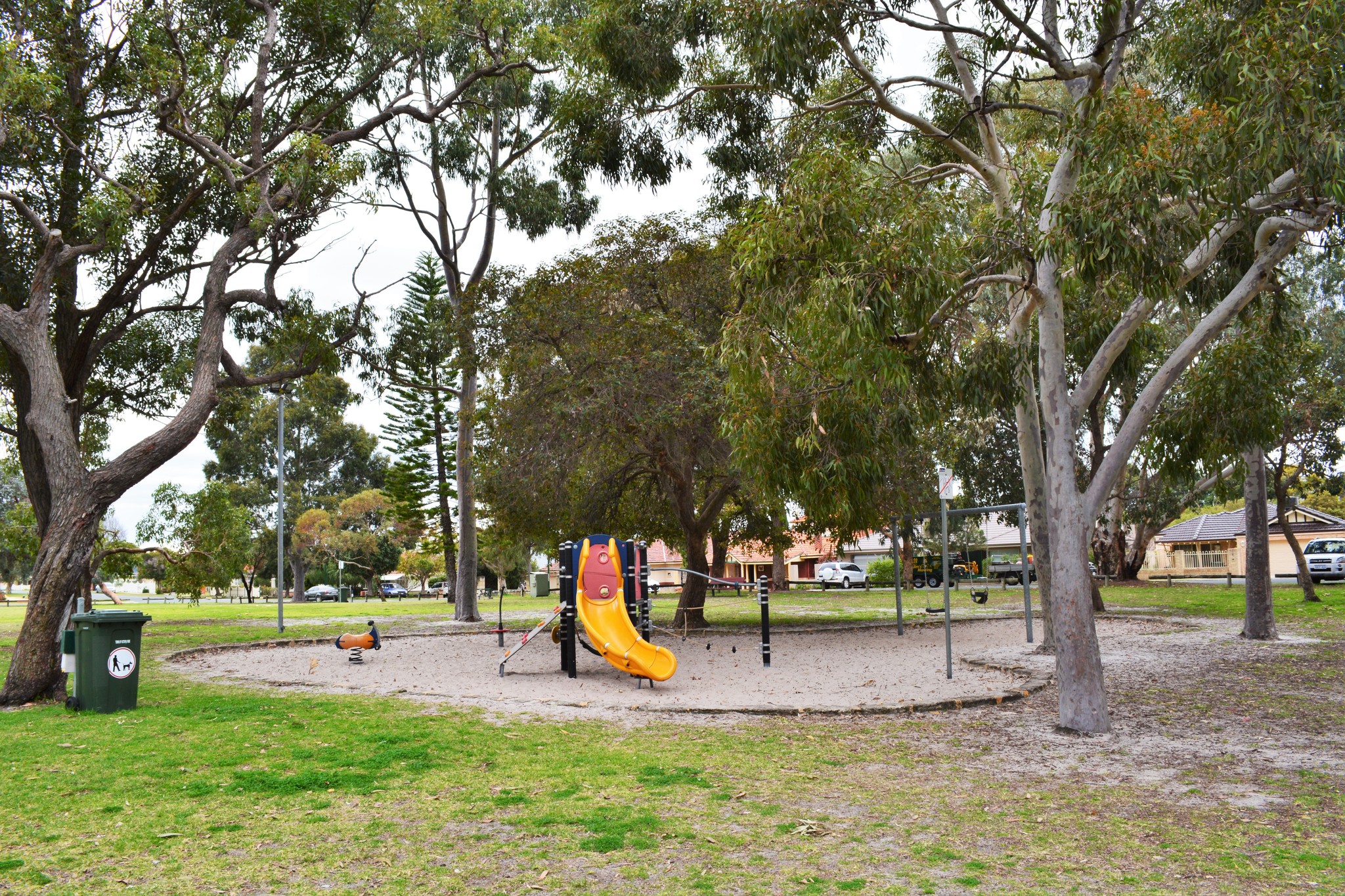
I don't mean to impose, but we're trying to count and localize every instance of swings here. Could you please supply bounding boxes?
[918,516,945,613]
[961,512,989,604]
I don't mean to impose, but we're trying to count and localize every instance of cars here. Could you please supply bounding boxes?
[377,583,408,598]
[304,585,338,603]
[95,582,116,593]
[647,577,661,594]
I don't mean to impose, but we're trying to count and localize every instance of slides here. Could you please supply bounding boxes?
[575,538,676,681]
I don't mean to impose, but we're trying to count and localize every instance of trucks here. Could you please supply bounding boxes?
[988,555,1038,587]
[911,552,961,588]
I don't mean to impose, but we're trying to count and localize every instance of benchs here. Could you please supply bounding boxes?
[708,577,745,593]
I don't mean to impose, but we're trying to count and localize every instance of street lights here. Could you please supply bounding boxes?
[269,381,295,633]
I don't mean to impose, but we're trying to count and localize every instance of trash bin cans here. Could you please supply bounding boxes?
[65,608,153,714]
[337,587,350,602]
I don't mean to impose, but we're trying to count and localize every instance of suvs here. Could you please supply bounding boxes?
[817,561,872,589]
[1296,536,1345,584]
[426,582,450,598]
